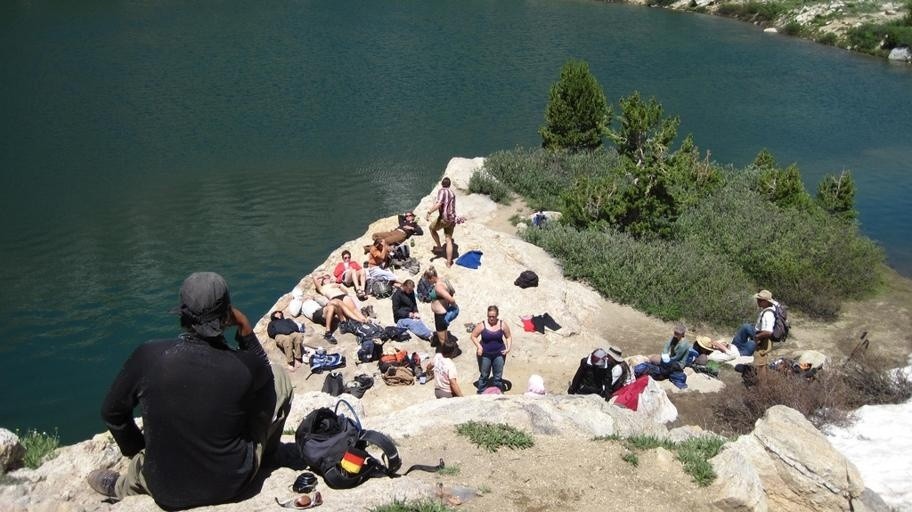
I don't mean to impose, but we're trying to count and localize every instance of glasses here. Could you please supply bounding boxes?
[405,214,412,217]
[274,491,324,511]
[488,316,496,318]
[274,311,282,313]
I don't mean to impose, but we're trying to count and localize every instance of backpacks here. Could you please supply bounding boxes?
[294,399,403,490]
[514,270,539,290]
[770,304,791,343]
[307,241,435,399]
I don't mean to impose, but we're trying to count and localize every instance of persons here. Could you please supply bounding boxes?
[267,178,513,398]
[568,290,788,394]
[100,271,295,511]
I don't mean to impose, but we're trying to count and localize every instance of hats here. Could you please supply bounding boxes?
[591,348,607,366]
[753,289,779,307]
[607,346,624,363]
[695,335,716,352]
[177,271,231,338]
[436,318,448,329]
[674,324,687,335]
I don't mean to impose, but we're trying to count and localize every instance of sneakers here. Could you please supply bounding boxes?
[86,469,121,498]
